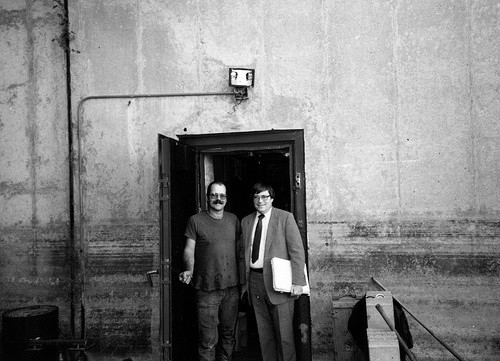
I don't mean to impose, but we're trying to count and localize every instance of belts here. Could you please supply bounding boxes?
[250,267,263,273]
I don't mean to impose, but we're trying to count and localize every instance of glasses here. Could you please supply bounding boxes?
[209,193,227,199]
[254,195,270,198]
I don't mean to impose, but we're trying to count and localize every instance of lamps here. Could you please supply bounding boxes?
[229,68,254,100]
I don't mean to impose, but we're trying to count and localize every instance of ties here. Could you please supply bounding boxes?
[252,213,265,264]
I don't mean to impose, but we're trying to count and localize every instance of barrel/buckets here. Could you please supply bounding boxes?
[2,306,59,361]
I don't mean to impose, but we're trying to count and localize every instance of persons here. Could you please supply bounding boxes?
[178,181,246,361]
[242,183,307,361]
